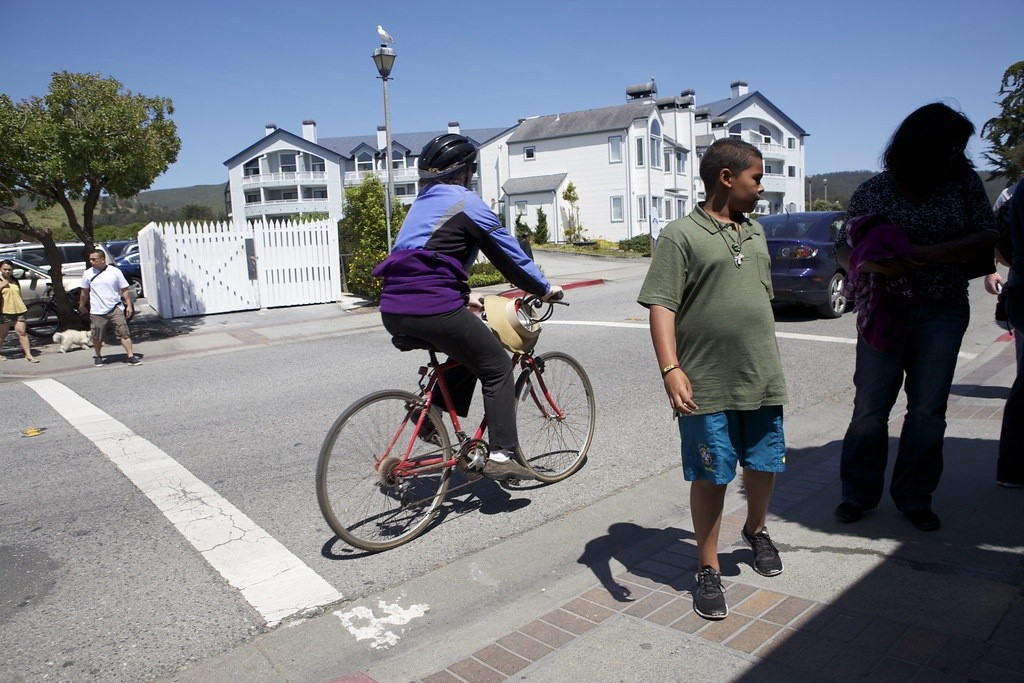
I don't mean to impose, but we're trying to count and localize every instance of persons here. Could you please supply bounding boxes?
[833,104,999,533]
[637,137,787,620]
[0,259,38,363]
[371,134,565,482]
[984,179,1024,487]
[78,249,143,366]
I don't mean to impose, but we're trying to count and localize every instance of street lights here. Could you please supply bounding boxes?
[370,43,399,256]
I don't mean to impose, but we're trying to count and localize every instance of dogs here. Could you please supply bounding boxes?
[53,330,92,354]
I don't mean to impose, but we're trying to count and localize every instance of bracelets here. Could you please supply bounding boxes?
[80,305,81,306]
[662,364,679,377]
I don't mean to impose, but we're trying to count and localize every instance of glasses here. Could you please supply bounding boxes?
[469,162,477,173]
[87,257,101,261]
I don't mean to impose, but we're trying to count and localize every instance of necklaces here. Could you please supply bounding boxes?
[702,204,745,268]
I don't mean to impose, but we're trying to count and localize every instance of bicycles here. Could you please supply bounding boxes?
[22,274,135,339]
[315,288,596,554]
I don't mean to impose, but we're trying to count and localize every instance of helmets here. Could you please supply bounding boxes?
[417,133,477,187]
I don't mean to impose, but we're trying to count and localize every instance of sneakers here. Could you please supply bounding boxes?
[741,518,785,577]
[127,355,143,366]
[692,564,729,620]
[92,355,104,367]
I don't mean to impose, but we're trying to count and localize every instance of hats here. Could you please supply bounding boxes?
[483,294,543,355]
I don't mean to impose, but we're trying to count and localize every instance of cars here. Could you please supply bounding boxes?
[0,237,143,328]
[749,209,858,318]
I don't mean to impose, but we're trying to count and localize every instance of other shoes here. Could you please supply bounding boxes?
[0,355,7,362]
[833,500,867,519]
[899,505,943,533]
[996,480,1024,488]
[23,356,40,363]
[482,458,537,481]
[410,408,445,447]
